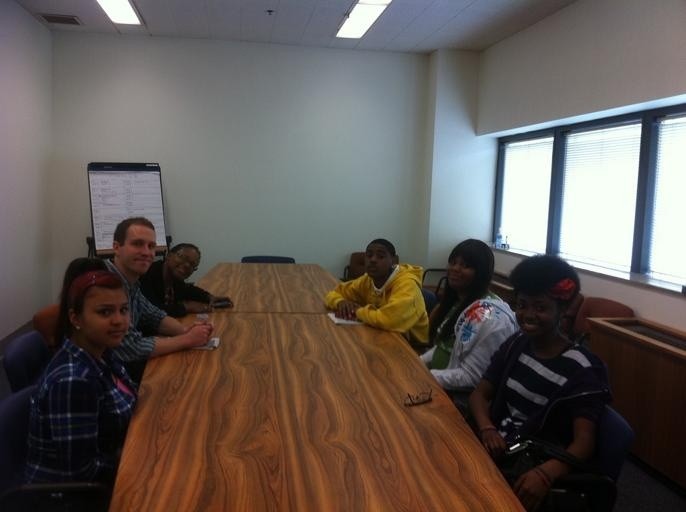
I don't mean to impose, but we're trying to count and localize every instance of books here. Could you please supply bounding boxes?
[328,311,364,325]
[192,338,220,350]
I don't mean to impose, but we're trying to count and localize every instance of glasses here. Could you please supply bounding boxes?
[404,389,433,406]
[176,252,198,271]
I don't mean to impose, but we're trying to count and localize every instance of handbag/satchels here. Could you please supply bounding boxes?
[496,440,618,512]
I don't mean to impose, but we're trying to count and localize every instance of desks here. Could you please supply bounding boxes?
[186,262,339,314]
[108,313,525,510]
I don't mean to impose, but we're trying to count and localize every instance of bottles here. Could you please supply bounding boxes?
[496,227,503,249]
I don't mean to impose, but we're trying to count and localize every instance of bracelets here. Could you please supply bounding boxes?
[480,425,496,432]
[538,466,555,484]
[533,466,552,489]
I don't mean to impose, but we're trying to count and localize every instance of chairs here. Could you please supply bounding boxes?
[520,401,637,512]
[0,304,104,511]
[343,253,365,279]
[573,295,633,344]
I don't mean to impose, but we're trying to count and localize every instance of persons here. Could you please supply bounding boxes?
[419,239,519,418]
[138,244,235,337]
[18,258,139,511]
[469,253,615,512]
[101,217,214,362]
[324,238,429,352]
